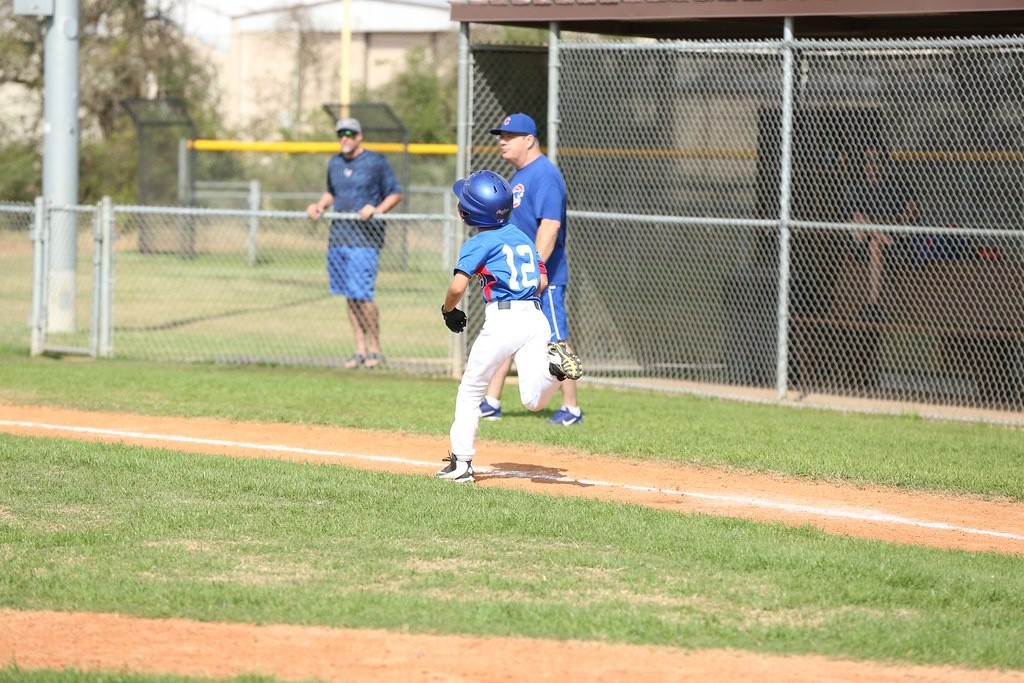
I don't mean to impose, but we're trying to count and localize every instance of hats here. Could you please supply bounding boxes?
[335,118,362,132]
[490,112,537,135]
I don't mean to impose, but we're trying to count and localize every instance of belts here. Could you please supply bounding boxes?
[498,300,540,309]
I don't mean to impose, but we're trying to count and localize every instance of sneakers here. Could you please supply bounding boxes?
[547,340,583,381]
[435,451,475,482]
[478,398,501,420]
[546,407,582,426]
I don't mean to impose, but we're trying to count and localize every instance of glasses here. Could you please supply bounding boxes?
[496,134,528,139]
[338,131,356,137]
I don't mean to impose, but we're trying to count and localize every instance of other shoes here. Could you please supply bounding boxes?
[344,354,363,369]
[363,353,383,368]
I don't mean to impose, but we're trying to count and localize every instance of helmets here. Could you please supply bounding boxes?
[453,170,514,228]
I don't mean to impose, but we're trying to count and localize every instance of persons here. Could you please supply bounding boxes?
[479,111,583,427]
[307,119,404,369]
[435,170,583,484]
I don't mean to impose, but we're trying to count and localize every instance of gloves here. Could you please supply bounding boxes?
[442,304,467,333]
